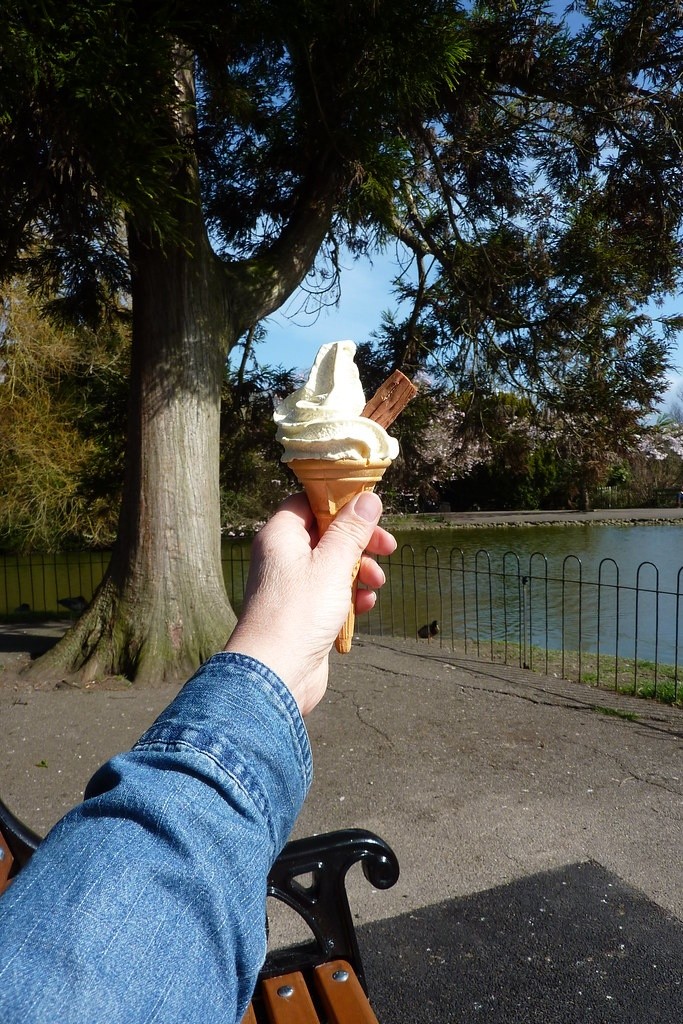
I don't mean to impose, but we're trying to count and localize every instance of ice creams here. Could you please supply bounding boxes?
[272,339,418,654]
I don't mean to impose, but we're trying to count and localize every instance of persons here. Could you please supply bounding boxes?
[0,488,399,1024]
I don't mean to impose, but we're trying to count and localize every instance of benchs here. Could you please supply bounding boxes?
[0,787,400,1024]
